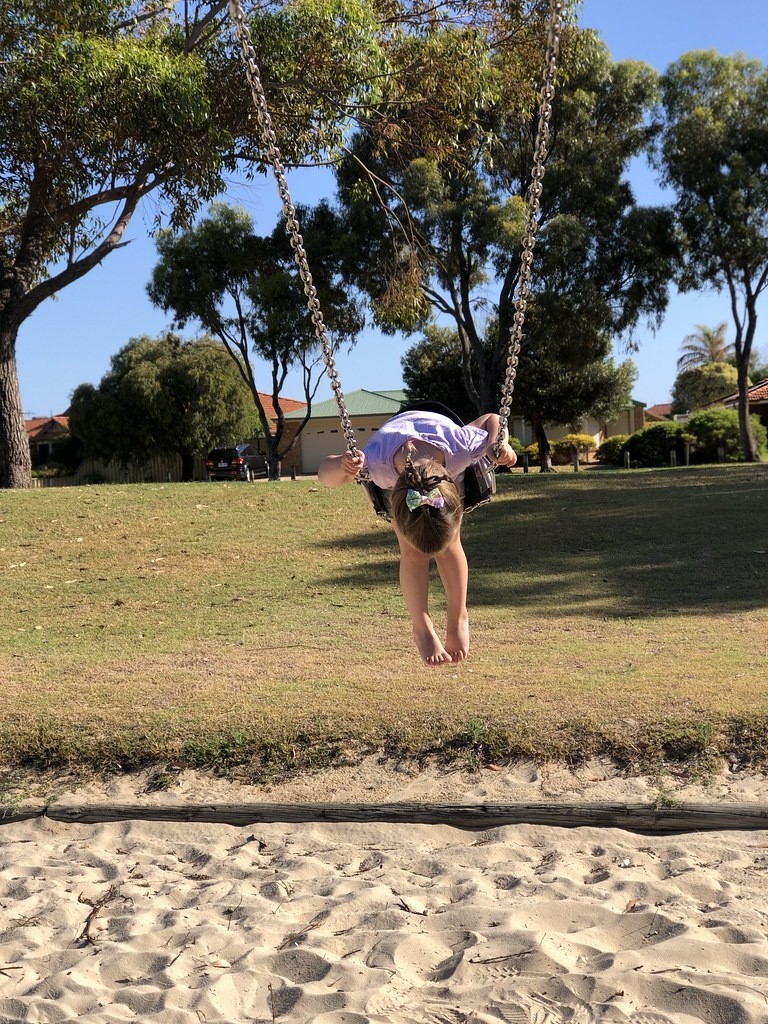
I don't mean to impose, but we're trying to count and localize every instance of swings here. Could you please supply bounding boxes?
[229,0,563,517]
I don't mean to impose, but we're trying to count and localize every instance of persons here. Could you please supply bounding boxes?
[317,410,517,665]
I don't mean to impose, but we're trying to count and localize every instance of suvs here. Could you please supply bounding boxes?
[206,443,269,484]
